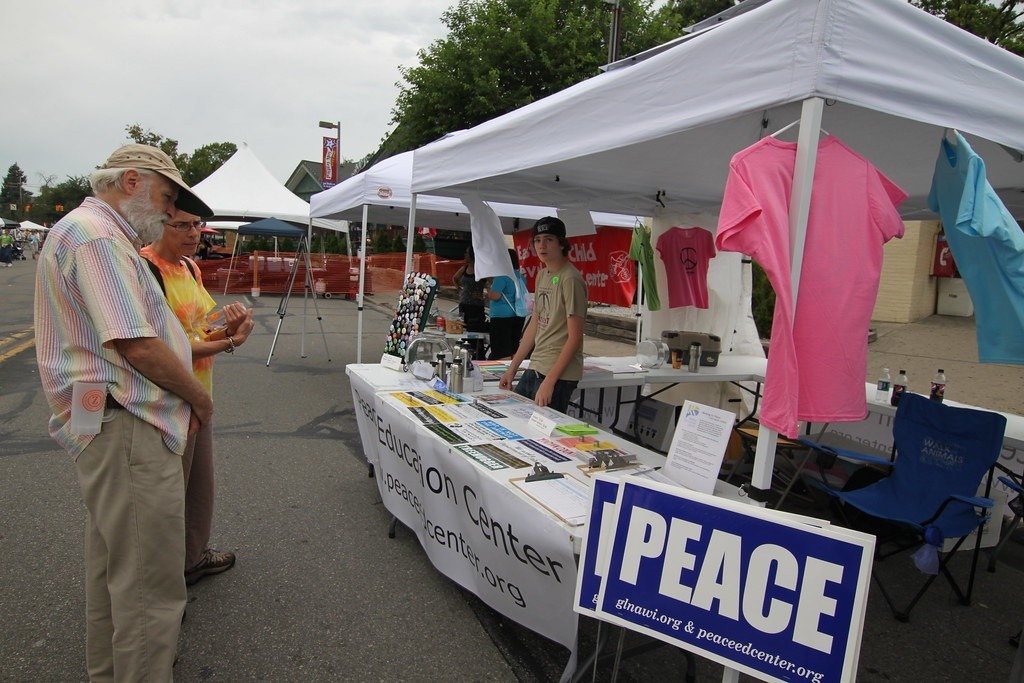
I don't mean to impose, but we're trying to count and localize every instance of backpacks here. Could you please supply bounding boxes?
[502,273,533,317]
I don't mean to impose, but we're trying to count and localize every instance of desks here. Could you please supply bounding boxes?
[344,353,1024,683]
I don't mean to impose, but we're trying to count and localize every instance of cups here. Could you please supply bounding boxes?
[671,348,684,369]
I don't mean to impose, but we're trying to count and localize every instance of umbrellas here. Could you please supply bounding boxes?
[0,217,21,229]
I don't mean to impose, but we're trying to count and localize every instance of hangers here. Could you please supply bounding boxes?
[633,216,642,228]
[768,118,829,143]
[941,128,959,142]
[675,215,698,230]
[475,180,490,207]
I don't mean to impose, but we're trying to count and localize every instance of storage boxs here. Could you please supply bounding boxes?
[936,276,974,318]
[660,330,720,367]
[871,214,940,323]
[936,486,1008,553]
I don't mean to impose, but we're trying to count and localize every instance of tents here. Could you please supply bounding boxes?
[189,0,1024,683]
[17,220,46,232]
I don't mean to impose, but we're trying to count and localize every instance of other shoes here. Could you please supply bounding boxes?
[0,262,12,267]
[31,251,40,259]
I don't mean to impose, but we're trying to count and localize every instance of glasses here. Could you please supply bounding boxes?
[164,219,206,232]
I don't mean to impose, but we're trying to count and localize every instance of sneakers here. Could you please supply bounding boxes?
[184,548,235,586]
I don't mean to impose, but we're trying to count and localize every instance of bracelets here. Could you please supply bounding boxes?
[226,327,229,338]
[224,336,236,355]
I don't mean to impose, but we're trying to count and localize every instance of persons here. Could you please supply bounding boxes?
[33,144,214,683]
[139,205,254,585]
[498,217,587,413]
[487,249,527,361]
[0,227,48,269]
[452,246,491,361]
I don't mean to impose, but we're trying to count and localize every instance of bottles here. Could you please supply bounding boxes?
[891,370,908,407]
[430,340,474,393]
[875,368,891,404]
[688,341,702,373]
[192,303,229,340]
[929,369,946,403]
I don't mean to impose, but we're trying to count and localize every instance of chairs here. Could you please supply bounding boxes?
[988,473,1024,575]
[822,392,1007,622]
[404,339,454,366]
[725,383,828,509]
[771,397,897,529]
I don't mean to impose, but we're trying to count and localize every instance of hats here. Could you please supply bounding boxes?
[534,216,566,237]
[101,144,214,218]
